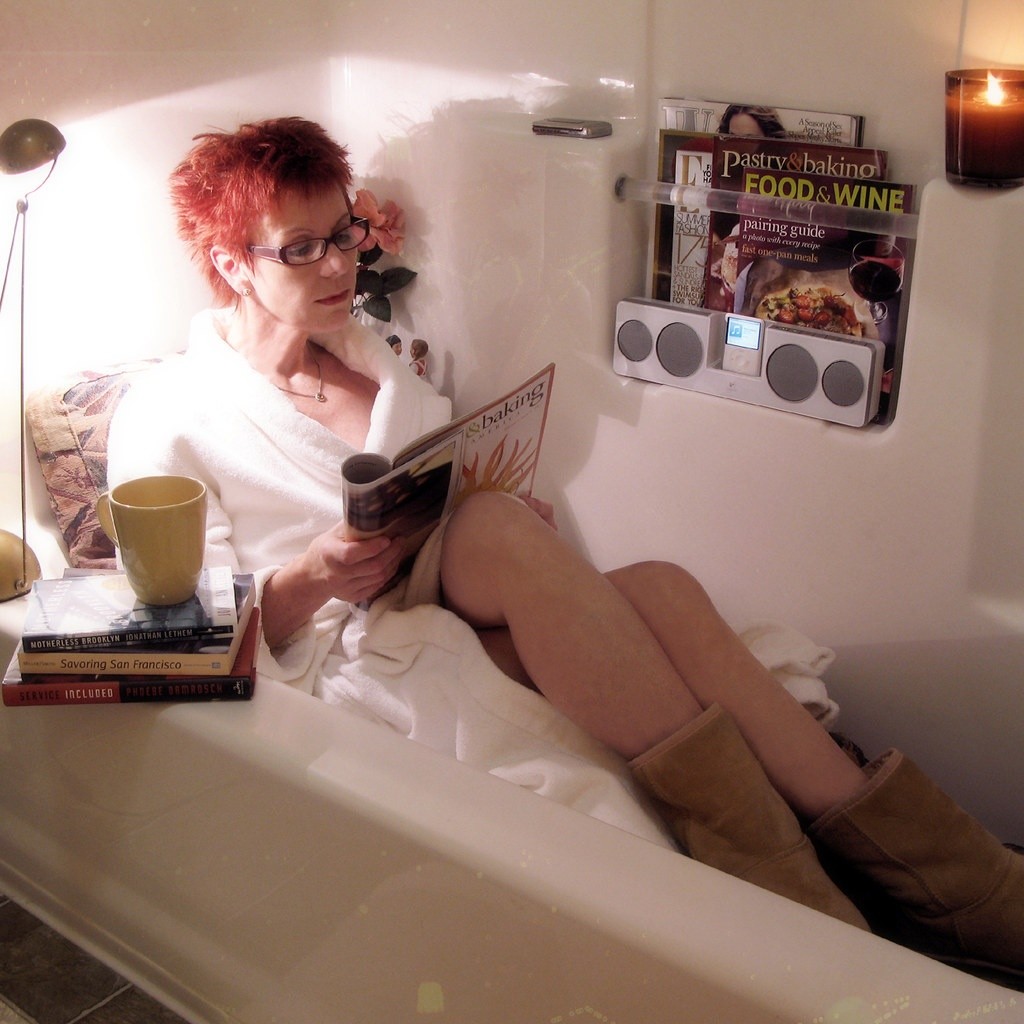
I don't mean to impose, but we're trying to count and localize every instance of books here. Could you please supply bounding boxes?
[2,569,261,702]
[341,362,553,615]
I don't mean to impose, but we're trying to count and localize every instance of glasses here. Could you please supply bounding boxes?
[248,215,370,266]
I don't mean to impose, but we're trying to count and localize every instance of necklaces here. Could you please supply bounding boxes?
[274,350,329,403]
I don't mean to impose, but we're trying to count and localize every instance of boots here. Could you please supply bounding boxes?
[628,704,870,933]
[806,748,1024,977]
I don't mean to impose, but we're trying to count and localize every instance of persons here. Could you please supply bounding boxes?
[105,118,1023,991]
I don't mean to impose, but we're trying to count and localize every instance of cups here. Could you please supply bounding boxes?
[96,474,208,605]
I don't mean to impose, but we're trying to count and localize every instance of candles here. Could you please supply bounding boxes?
[945,69,1024,189]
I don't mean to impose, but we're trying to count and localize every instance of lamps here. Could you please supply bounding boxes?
[0,118,66,603]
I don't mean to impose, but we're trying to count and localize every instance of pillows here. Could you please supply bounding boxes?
[27,348,189,569]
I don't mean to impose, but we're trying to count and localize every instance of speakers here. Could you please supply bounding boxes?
[611,297,885,426]
